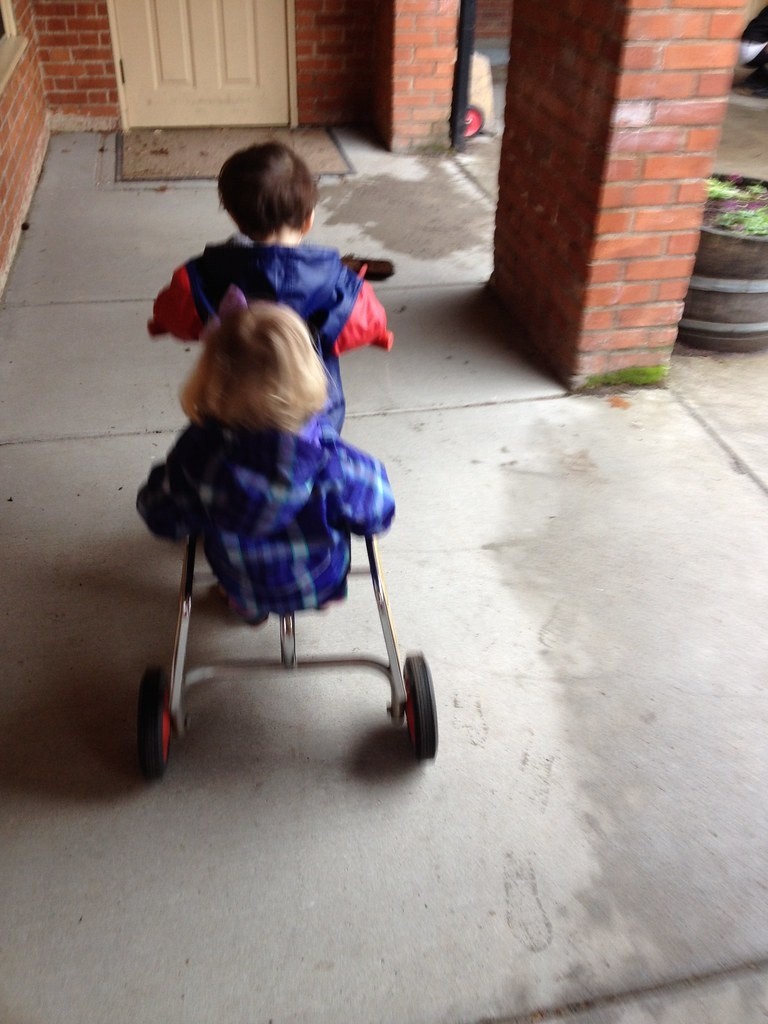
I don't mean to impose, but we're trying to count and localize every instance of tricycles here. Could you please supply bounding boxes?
[134,256,440,774]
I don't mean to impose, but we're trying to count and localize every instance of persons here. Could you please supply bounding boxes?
[146,140,392,431]
[133,299,397,626]
